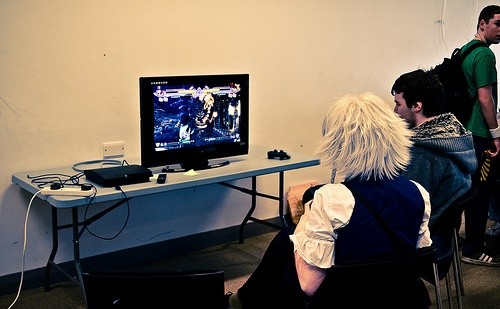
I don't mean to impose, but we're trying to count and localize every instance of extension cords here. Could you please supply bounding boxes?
[41,186,95,197]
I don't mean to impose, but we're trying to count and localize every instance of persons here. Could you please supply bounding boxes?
[457,4,500,265]
[224,93,433,309]
[389,69,478,280]
[171,83,242,142]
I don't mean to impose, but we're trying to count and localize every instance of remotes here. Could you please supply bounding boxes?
[157,174,167,184]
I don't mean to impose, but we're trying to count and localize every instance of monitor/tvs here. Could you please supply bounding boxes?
[137,74,249,173]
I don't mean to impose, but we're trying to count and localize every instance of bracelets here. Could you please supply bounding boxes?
[489,126,500,138]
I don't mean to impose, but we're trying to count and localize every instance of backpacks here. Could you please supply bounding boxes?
[426,43,489,134]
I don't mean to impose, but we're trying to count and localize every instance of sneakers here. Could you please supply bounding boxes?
[460,246,500,266]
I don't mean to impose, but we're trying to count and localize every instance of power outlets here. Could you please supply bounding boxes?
[104,142,125,156]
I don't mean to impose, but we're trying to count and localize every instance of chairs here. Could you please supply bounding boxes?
[82,272,233,309]
[437,184,476,309]
[327,249,443,309]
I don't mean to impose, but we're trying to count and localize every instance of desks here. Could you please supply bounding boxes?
[12,143,320,309]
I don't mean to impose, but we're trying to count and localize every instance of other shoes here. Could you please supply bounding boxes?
[488,221,500,236]
